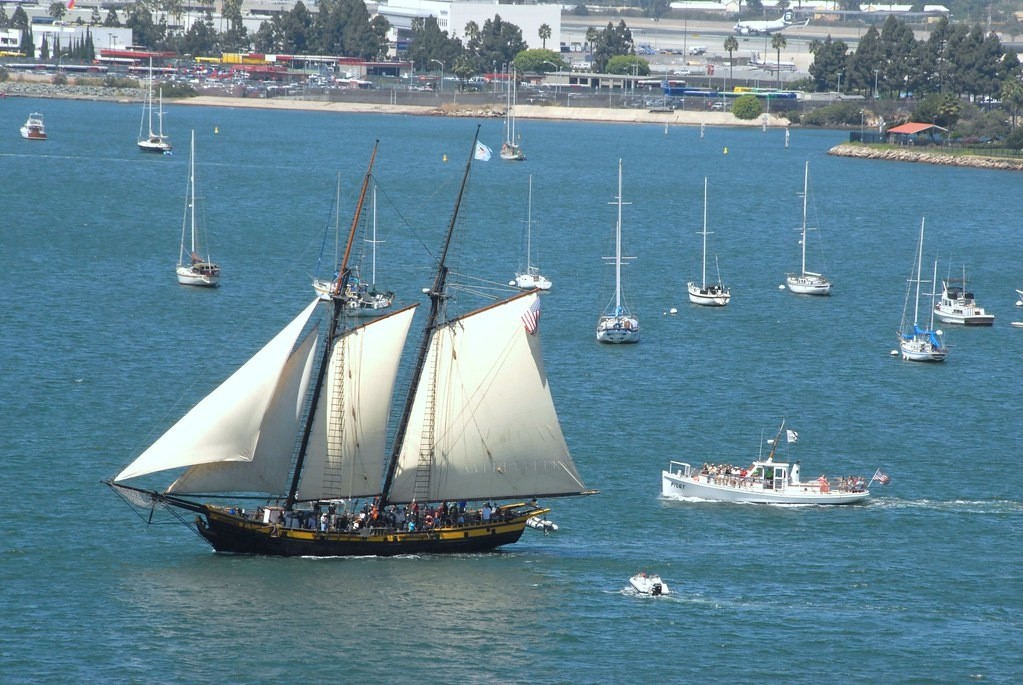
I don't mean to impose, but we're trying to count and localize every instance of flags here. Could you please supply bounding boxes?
[474,140,493,161]
[873,471,890,485]
[787,430,798,442]
[520,297,541,335]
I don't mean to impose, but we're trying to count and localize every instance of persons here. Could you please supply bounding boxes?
[690,463,760,488]
[651,575,661,584]
[834,475,867,493]
[227,507,242,517]
[817,474,828,492]
[482,502,513,520]
[278,501,468,531]
[764,468,773,489]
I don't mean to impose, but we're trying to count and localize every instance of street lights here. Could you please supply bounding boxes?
[860,109,864,142]
[836,73,843,101]
[60,53,68,72]
[409,61,416,91]
[431,60,443,92]
[108,34,117,72]
[874,70,879,97]
[544,60,557,103]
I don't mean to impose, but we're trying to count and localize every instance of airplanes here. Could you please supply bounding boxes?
[733,10,809,35]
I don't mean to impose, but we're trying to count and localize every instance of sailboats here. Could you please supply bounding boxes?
[597,158,639,343]
[499,68,526,160]
[99,125,601,555]
[137,57,172,151]
[516,174,553,291]
[312,170,394,317]
[174,129,220,287]
[785,161,833,295]
[687,177,731,307]
[895,217,951,361]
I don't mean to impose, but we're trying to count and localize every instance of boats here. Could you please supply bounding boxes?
[934,255,995,326]
[20,112,48,140]
[662,418,890,503]
[629,572,670,597]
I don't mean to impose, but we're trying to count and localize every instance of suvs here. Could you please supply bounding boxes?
[673,69,690,76]
